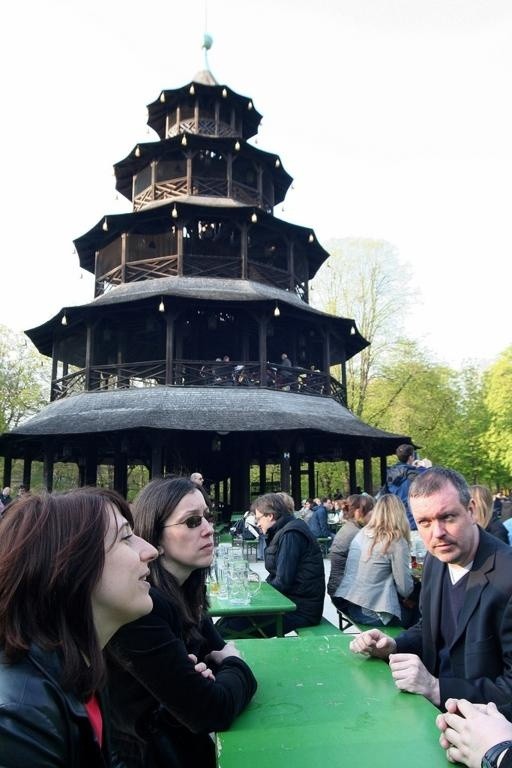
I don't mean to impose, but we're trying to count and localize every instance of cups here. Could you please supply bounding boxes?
[227,561,260,604]
[205,543,231,600]
[229,547,243,561]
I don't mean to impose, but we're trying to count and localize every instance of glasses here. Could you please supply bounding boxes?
[256,515,264,521]
[163,513,215,528]
[194,476,202,481]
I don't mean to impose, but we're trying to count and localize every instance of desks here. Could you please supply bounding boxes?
[214,635,469,768]
[205,573,296,638]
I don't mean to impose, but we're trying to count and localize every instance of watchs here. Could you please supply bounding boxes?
[480,741,511,768]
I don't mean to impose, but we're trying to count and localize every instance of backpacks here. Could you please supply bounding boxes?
[230,517,245,537]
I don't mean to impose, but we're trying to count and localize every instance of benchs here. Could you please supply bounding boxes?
[244,539,259,560]
[339,610,410,641]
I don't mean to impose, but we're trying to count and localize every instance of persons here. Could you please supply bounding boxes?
[105,476,257,733]
[243,503,268,560]
[0,486,12,511]
[349,466,512,724]
[436,696,511,766]
[213,493,326,638]
[0,487,217,768]
[327,495,376,602]
[212,355,234,384]
[469,485,509,546]
[297,496,347,557]
[386,443,432,530]
[274,353,291,387]
[190,473,204,487]
[332,494,422,629]
[16,485,25,499]
